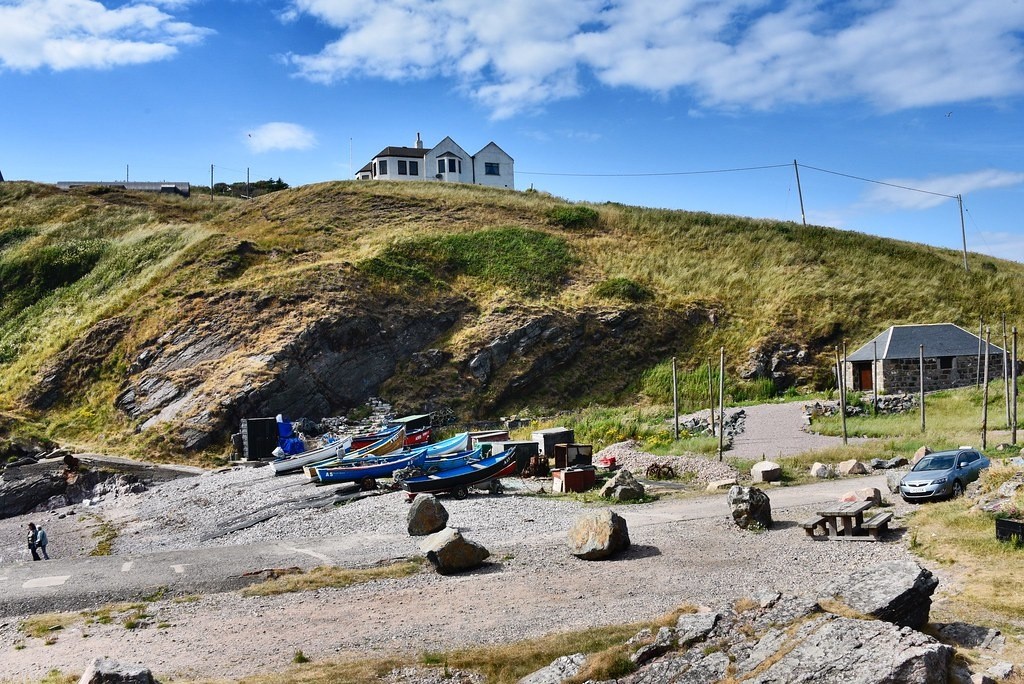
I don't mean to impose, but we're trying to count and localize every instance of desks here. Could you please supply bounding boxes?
[816,500,873,542]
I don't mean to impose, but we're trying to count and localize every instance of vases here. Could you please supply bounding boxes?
[996,517,1024,542]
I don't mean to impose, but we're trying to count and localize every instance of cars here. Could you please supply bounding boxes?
[898,449,990,504]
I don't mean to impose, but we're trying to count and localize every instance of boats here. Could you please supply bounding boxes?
[398,445,519,503]
[268,437,352,477]
[302,425,407,482]
[407,431,469,456]
[315,448,430,486]
[351,425,432,450]
[423,446,483,470]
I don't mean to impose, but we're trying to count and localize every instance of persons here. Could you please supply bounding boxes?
[27,522,49,561]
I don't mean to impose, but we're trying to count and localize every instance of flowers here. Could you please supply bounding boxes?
[994,507,1024,520]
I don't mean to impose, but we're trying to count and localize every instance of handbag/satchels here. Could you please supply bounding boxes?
[36,541,42,547]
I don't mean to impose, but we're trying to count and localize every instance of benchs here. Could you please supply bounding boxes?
[798,515,829,541]
[860,513,894,541]
